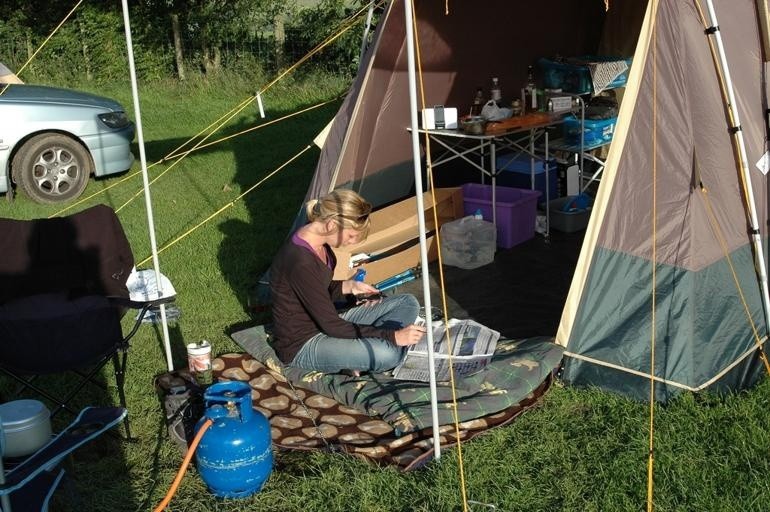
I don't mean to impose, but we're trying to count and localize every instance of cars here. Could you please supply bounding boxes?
[0,83,136,207]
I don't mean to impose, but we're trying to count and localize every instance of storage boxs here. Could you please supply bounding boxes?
[495,152,558,210]
[460,182,543,249]
[562,115,618,149]
[541,56,631,93]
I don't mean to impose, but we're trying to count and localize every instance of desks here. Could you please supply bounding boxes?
[407,107,564,257]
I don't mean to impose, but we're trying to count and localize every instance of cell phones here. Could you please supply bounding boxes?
[357,290,383,301]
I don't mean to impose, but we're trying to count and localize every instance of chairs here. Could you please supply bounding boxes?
[0,408,128,512]
[0,204,178,443]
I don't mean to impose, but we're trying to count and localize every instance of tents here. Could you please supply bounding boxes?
[285,0,770,400]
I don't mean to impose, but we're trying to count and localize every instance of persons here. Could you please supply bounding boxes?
[266,187,432,377]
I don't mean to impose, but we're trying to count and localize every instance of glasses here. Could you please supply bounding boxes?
[339,213,369,223]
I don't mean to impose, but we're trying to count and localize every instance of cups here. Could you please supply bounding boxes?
[187,341,213,388]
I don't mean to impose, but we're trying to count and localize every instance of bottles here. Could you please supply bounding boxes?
[525,65,538,113]
[489,77,502,108]
[535,84,545,112]
[547,99,553,112]
[473,88,484,115]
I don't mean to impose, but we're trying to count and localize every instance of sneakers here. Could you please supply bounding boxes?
[155,374,204,444]
[420,305,442,320]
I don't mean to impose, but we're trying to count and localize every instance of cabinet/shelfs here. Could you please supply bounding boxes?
[528,82,625,247]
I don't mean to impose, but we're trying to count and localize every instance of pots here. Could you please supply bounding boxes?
[0,399,53,459]
[461,116,503,135]
